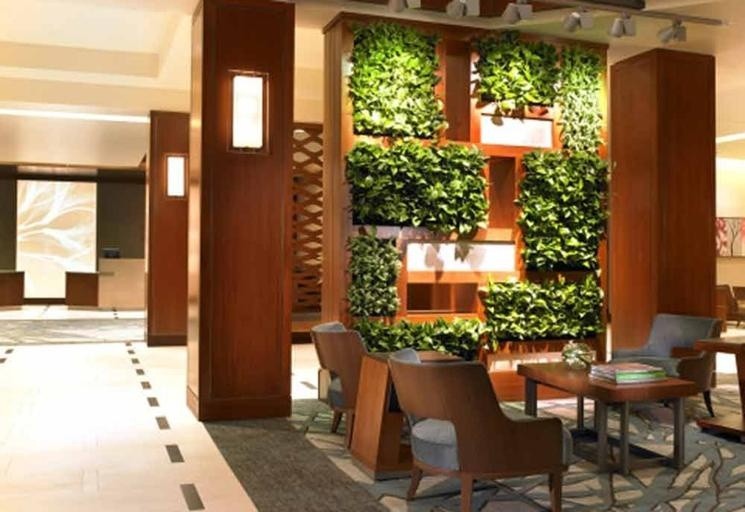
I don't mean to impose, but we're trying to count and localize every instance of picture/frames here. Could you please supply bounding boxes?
[715,217,745,258]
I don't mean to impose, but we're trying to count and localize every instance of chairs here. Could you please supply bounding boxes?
[613,313,724,418]
[716,284,738,311]
[732,286,745,301]
[301,321,368,448]
[386,349,572,512]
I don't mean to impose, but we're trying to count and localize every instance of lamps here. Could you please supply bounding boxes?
[389,0,722,43]
[162,152,189,202]
[225,69,270,158]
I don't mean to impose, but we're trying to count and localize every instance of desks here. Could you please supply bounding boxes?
[695,337,745,442]
[350,351,466,481]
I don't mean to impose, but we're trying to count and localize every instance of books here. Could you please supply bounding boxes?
[586,363,670,385]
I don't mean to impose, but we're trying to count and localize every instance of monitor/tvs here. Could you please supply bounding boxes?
[101,247,121,258]
